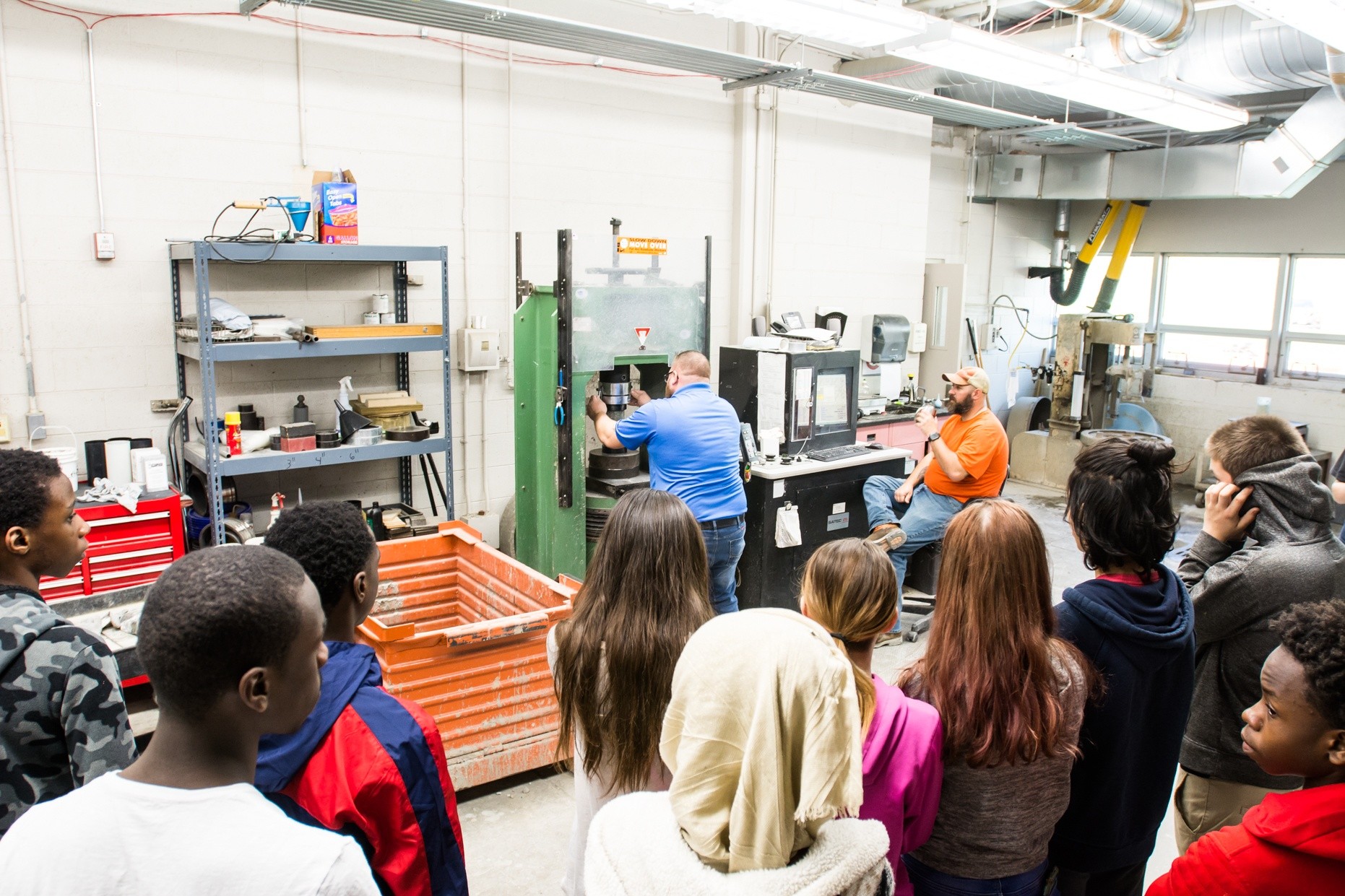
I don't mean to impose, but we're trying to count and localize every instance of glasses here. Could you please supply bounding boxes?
[663,371,678,382]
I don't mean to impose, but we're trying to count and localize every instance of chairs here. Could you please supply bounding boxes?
[904,464,1011,642]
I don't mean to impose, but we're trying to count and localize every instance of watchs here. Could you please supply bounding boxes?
[927,431,942,441]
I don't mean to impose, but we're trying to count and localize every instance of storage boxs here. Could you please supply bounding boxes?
[311,169,358,245]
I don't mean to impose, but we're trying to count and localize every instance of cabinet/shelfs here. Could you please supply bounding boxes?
[167,244,454,549]
[854,416,952,459]
[718,346,912,614]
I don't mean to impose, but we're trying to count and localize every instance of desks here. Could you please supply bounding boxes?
[39,478,192,603]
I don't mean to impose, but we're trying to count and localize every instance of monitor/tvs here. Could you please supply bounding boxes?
[815,366,850,437]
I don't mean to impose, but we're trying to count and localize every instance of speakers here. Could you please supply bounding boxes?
[752,316,766,337]
[827,318,841,344]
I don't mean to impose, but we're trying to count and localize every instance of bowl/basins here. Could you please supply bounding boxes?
[1006,396,1051,465]
[1155,366,1161,374]
[1107,403,1166,436]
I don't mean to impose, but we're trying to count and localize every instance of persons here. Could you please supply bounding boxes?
[585,607,899,896]
[547,489,721,896]
[586,350,749,616]
[0,545,382,896]
[797,538,944,896]
[864,366,1008,649]
[1329,450,1345,542]
[1144,598,1345,896]
[248,498,469,896]
[1043,435,1197,896]
[1172,416,1345,852]
[0,445,140,839]
[890,499,1089,896]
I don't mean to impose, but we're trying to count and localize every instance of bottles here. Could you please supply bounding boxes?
[935,395,942,408]
[294,395,309,423]
[225,412,241,455]
[366,502,384,541]
[907,375,914,391]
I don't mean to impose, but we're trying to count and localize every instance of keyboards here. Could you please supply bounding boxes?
[807,445,871,462]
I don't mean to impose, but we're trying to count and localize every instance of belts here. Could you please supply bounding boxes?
[698,513,745,530]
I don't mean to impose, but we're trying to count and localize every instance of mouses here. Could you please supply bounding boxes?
[866,443,883,450]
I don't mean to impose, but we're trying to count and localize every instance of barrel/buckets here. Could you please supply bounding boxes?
[187,501,252,552]
[30,426,78,492]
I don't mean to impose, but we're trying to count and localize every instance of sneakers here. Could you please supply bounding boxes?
[873,632,902,648]
[863,523,907,553]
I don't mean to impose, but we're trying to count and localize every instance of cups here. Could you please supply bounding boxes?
[1256,369,1266,384]
[85,438,152,487]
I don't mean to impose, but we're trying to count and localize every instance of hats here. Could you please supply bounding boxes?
[942,366,989,394]
